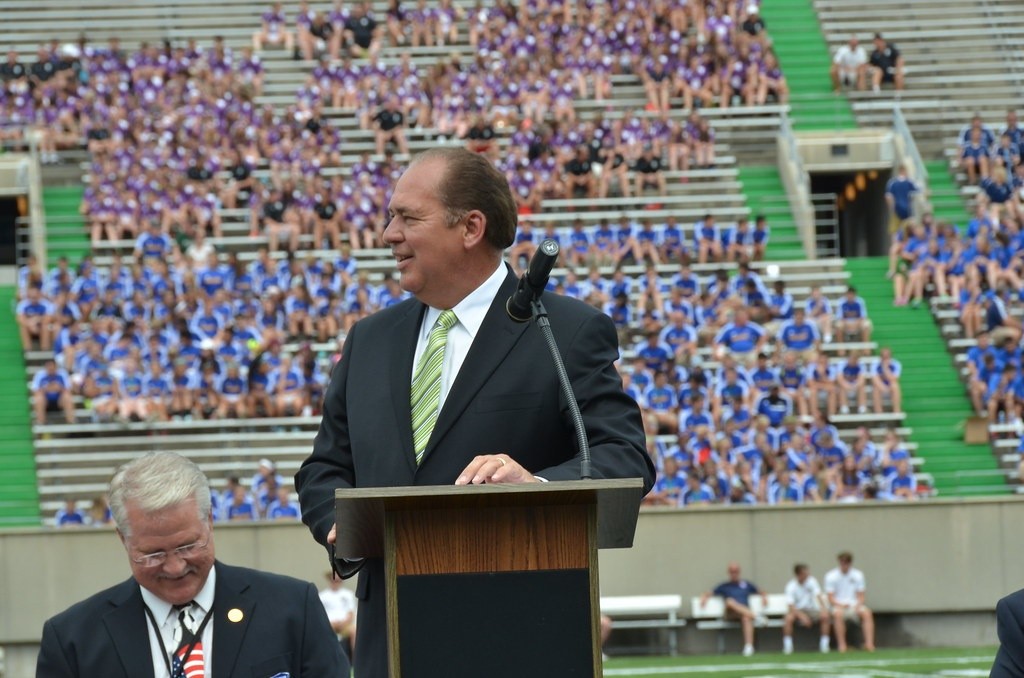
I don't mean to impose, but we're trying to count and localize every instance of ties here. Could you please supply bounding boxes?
[409,309,459,469]
[171,605,205,678]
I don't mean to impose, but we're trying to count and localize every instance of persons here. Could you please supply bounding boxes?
[890,190,1024,341]
[955,106,1024,233]
[643,409,923,506]
[0,0,788,257]
[828,32,869,92]
[14,240,408,434]
[885,162,918,230]
[54,494,84,526]
[208,458,300,521]
[966,319,1024,489]
[543,257,901,430]
[35,450,353,678]
[294,146,657,579]
[988,587,1024,678]
[867,29,905,101]
[783,564,830,654]
[699,562,768,656]
[84,498,113,524]
[824,549,874,654]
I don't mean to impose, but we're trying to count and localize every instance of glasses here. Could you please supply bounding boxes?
[121,517,210,568]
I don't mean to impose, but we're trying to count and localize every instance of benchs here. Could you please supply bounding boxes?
[0,0,1024,526]
[689,593,860,654]
[599,594,687,657]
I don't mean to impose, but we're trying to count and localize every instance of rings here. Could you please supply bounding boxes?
[497,457,506,464]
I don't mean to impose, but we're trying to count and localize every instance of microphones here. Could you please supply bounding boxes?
[506,237,560,323]
